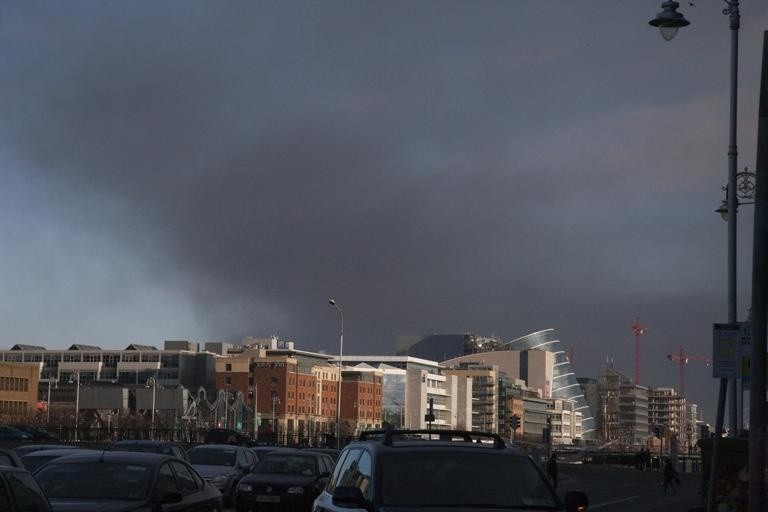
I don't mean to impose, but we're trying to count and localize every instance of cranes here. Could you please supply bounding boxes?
[666,349,712,393]
[632,316,647,386]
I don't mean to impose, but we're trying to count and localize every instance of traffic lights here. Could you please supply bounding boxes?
[515,416,520,427]
[510,417,514,427]
[653,425,660,438]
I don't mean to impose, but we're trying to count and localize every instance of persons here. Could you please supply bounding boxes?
[644,448,653,473]
[662,458,678,495]
[637,447,647,471]
[546,452,558,491]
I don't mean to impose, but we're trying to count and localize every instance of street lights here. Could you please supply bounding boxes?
[328,299,345,447]
[46,372,59,424]
[642,0,751,510]
[144,376,156,440]
[69,369,80,442]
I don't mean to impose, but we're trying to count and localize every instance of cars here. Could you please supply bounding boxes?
[1,448,52,512]
[234,450,336,512]
[1,426,297,499]
[29,451,223,512]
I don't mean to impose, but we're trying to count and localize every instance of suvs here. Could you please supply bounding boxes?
[309,428,591,512]
